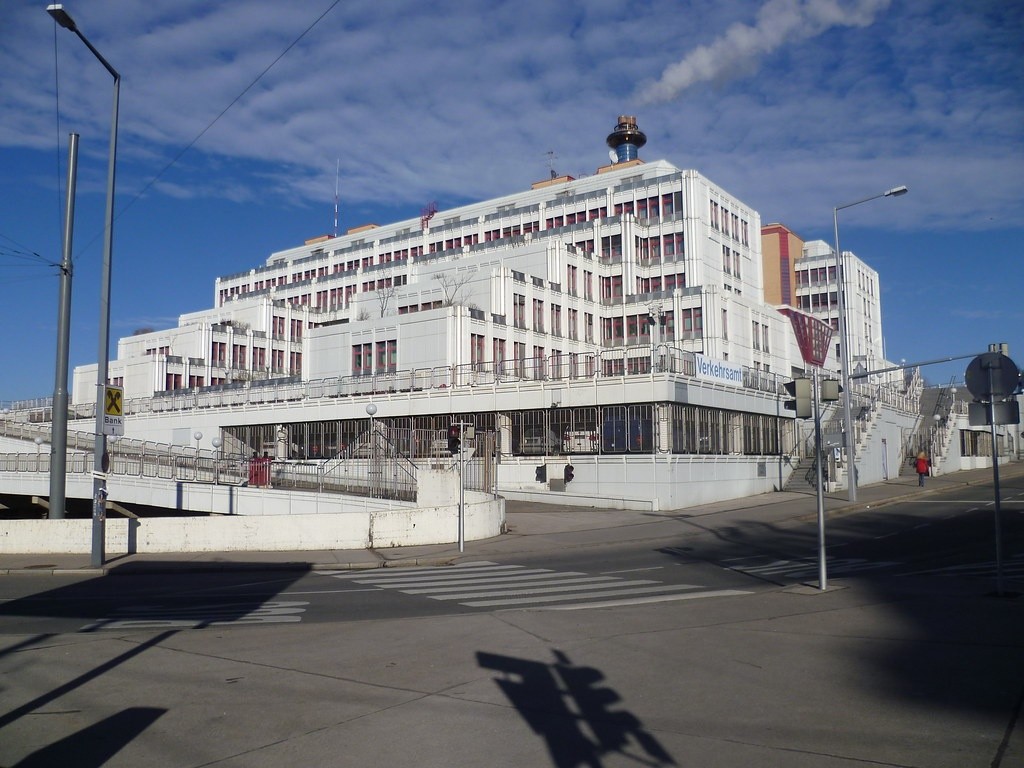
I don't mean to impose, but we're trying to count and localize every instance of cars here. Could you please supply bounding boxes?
[260,414,651,458]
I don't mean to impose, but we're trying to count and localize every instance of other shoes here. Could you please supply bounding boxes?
[919,481,924,487]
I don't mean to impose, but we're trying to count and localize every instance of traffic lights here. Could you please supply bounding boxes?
[565,464,574,482]
[820,378,844,403]
[785,379,813,420]
[448,427,459,455]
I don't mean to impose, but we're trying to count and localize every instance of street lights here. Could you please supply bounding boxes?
[3,408,10,429]
[107,435,118,472]
[193,431,203,473]
[831,185,911,501]
[35,437,44,471]
[46,5,122,564]
[213,436,223,485]
[366,404,378,494]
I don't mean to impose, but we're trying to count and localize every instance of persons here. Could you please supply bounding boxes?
[914,452,929,488]
